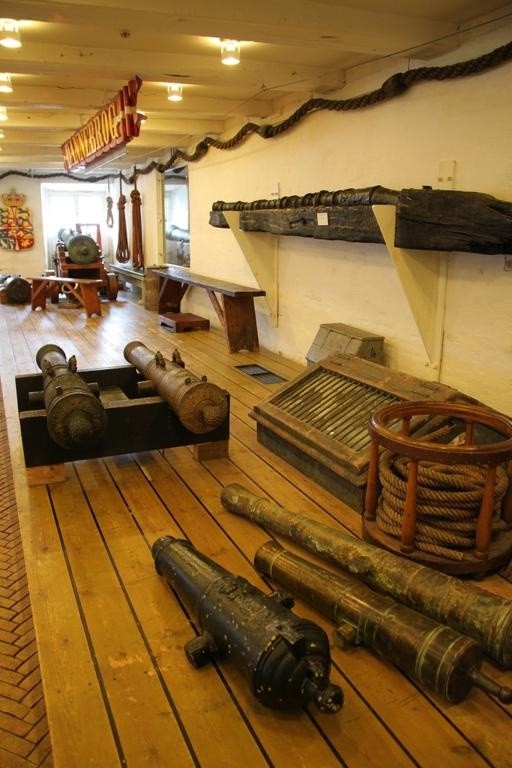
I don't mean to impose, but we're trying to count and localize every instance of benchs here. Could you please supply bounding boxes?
[28,275,103,317]
[151,265,268,355]
